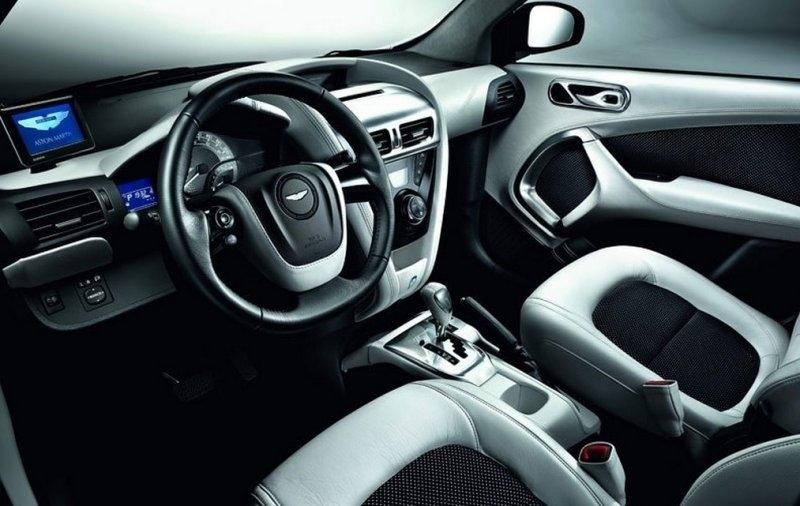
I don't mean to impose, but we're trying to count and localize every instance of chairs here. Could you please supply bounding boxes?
[516,243,799,474]
[246,373,797,506]
